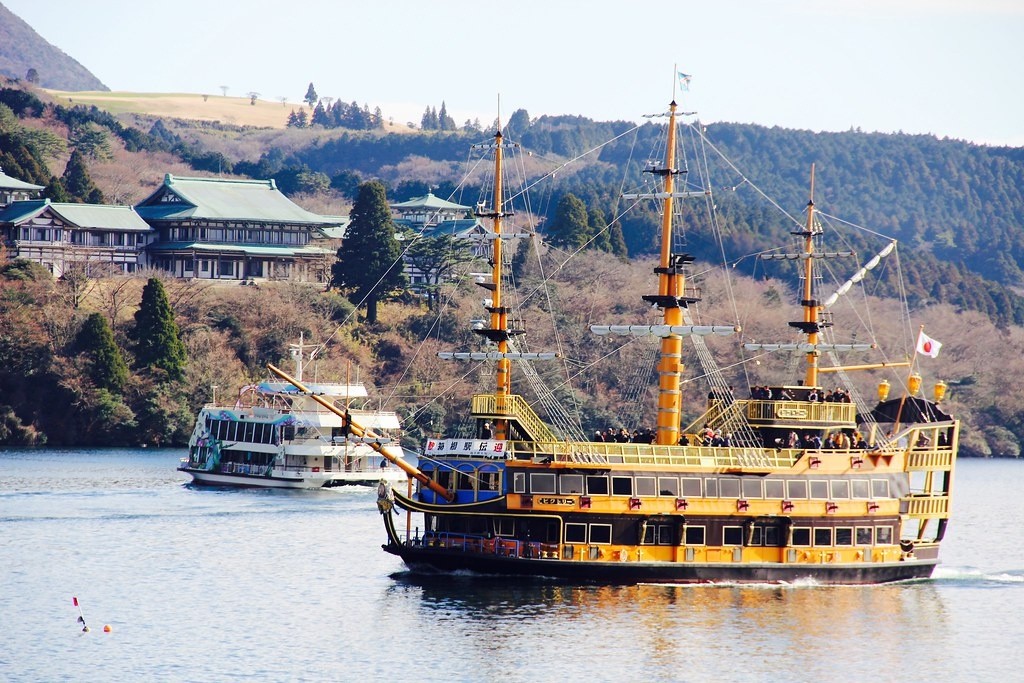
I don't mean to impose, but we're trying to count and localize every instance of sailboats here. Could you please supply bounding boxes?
[254,61,962,586]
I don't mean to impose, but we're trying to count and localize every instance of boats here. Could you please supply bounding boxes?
[176,330,407,492]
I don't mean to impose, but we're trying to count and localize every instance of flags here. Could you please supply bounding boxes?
[916,332,942,358]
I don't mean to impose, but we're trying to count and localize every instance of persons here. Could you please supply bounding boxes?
[677,427,764,448]
[777,431,821,452]
[594,427,656,444]
[916,432,930,447]
[707,384,852,425]
[938,432,947,445]
[380,458,387,467]
[825,431,907,450]
